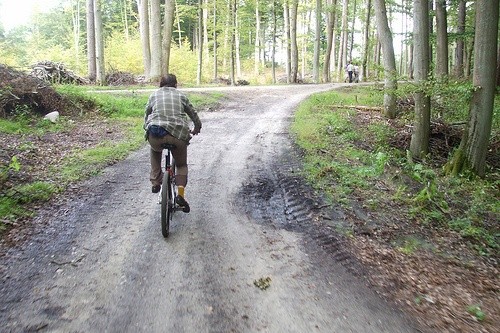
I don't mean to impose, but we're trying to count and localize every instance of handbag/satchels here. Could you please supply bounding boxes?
[149,125,168,137]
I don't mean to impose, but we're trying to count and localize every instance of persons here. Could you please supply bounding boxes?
[143,73,202,213]
[344,62,359,84]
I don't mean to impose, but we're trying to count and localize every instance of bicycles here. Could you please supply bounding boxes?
[152,130,197,237]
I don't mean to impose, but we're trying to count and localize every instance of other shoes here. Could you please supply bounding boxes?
[152,185,161,193]
[175,195,190,213]
